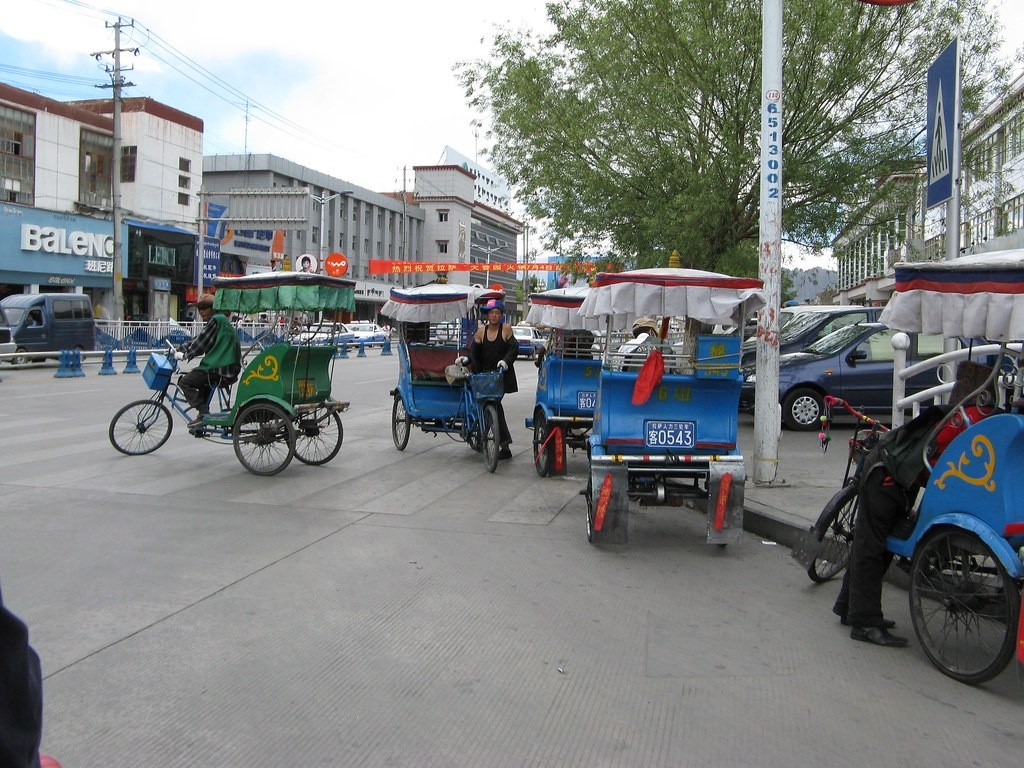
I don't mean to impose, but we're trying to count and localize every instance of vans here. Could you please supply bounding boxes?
[0,293,95,364]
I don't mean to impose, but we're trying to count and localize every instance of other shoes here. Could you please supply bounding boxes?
[188,416,204,429]
[477,438,487,450]
[498,449,513,459]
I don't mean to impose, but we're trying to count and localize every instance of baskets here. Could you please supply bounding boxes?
[849,430,887,465]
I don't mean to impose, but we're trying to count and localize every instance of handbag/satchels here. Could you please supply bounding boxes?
[949,337,996,406]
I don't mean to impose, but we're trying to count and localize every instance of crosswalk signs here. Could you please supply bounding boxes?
[926,37,954,209]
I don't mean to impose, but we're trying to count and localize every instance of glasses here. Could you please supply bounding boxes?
[198,305,213,311]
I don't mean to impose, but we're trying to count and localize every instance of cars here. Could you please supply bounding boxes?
[739,323,1014,431]
[291,322,355,352]
[741,304,883,366]
[510,321,546,361]
[350,320,385,347]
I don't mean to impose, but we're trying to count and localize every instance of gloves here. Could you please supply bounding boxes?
[165,348,178,355]
[174,352,186,361]
[455,356,469,364]
[497,360,508,371]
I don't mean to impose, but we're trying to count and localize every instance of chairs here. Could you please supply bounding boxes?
[407,344,470,378]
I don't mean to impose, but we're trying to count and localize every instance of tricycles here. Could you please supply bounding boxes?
[525,288,605,478]
[575,268,767,546]
[109,271,358,476]
[789,248,1023,684]
[381,276,503,473]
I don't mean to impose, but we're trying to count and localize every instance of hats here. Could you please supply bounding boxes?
[631,318,659,337]
[480,301,505,314]
[193,294,215,306]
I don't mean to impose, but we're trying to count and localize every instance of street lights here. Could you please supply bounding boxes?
[309,191,353,274]
[471,244,508,289]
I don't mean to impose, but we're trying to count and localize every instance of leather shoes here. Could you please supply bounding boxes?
[841,612,895,626]
[852,626,908,646]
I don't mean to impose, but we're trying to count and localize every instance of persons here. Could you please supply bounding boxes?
[831,405,954,648]
[299,257,314,275]
[535,337,556,367]
[382,322,392,337]
[28,312,37,326]
[165,294,241,438]
[277,317,285,328]
[455,299,518,459]
[259,314,269,327]
[564,328,595,359]
[615,315,677,373]
